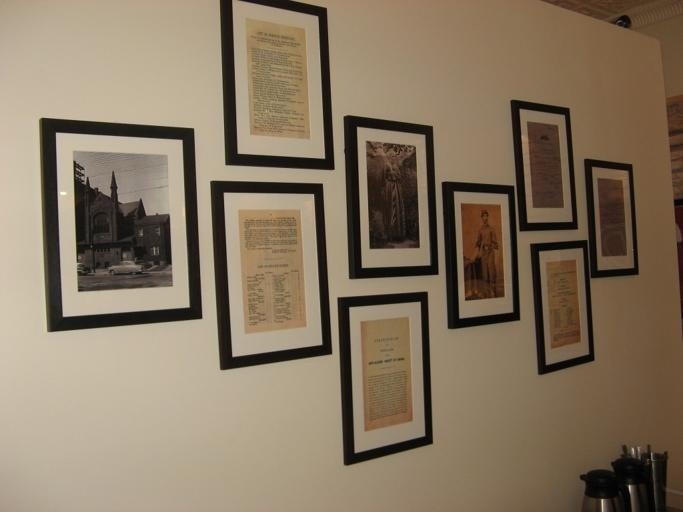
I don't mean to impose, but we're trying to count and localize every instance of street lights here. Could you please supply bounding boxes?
[90,240,95,273]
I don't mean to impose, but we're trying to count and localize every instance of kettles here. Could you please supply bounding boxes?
[581,458,655,512]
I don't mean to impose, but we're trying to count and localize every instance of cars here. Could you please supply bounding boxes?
[77,263,89,275]
[108,260,143,275]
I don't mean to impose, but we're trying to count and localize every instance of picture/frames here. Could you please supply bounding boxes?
[441,173,523,330]
[342,111,444,281]
[41,112,201,324]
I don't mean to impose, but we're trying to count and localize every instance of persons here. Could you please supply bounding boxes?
[475,210,499,297]
